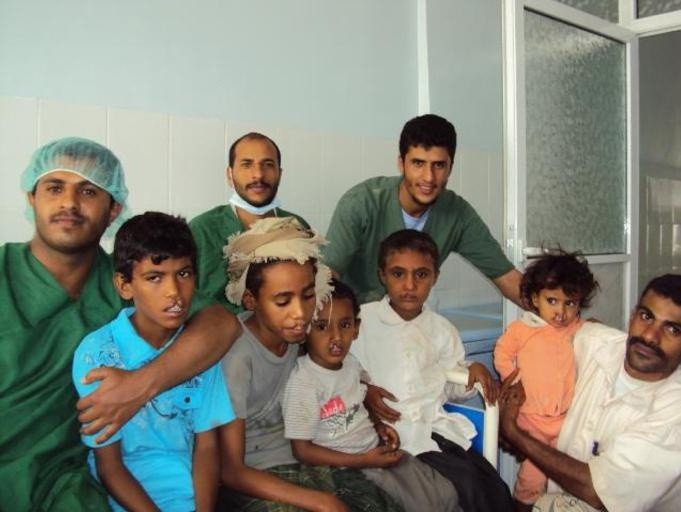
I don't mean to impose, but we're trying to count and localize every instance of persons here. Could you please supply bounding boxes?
[184,131,314,319]
[280,278,460,511]
[215,215,402,512]
[501,273,681,512]
[1,136,243,511]
[70,210,238,511]
[350,229,512,512]
[493,244,602,512]
[322,113,532,314]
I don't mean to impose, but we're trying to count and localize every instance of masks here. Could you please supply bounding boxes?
[228,185,281,216]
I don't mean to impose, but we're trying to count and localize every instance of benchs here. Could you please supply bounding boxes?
[440,366,504,482]
